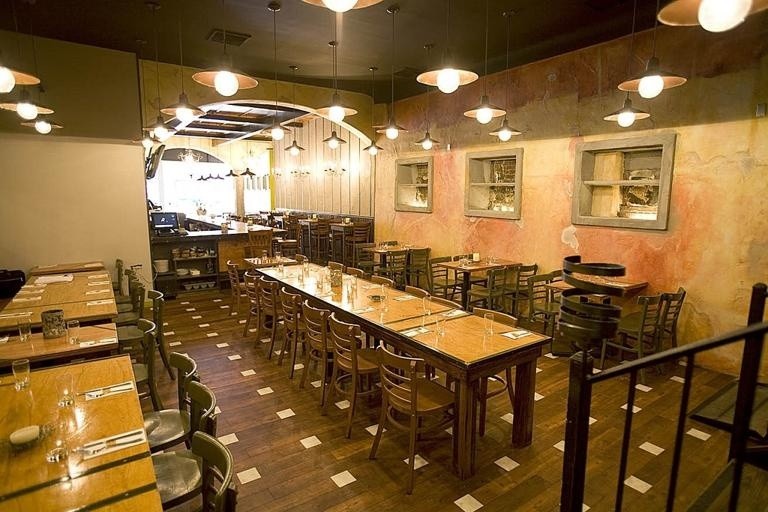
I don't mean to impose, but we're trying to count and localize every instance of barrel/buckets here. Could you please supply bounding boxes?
[154,260,169,273]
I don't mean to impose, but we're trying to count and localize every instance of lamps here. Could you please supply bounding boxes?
[302,0,381,13]
[159,90,206,122]
[362,139,383,155]
[603,96,651,127]
[132,128,162,148]
[284,138,306,157]
[1,87,56,120]
[260,114,292,141]
[190,50,260,97]
[416,43,479,93]
[413,127,441,149]
[142,116,177,139]
[374,115,409,140]
[488,117,522,142]
[463,90,506,124]
[657,1,767,33]
[618,54,688,98]
[1,62,41,97]
[19,113,65,133]
[178,149,349,182]
[321,130,348,148]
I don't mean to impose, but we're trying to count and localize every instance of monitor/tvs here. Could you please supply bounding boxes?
[151,212,179,235]
[151,145,165,178]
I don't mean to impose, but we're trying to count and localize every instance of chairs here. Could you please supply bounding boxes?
[223,209,686,381]
[225,251,552,494]
[0,257,239,511]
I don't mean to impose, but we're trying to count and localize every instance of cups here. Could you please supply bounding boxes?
[256,248,499,311]
[484,312,494,330]
[436,315,447,335]
[0,309,145,464]
[421,295,433,317]
